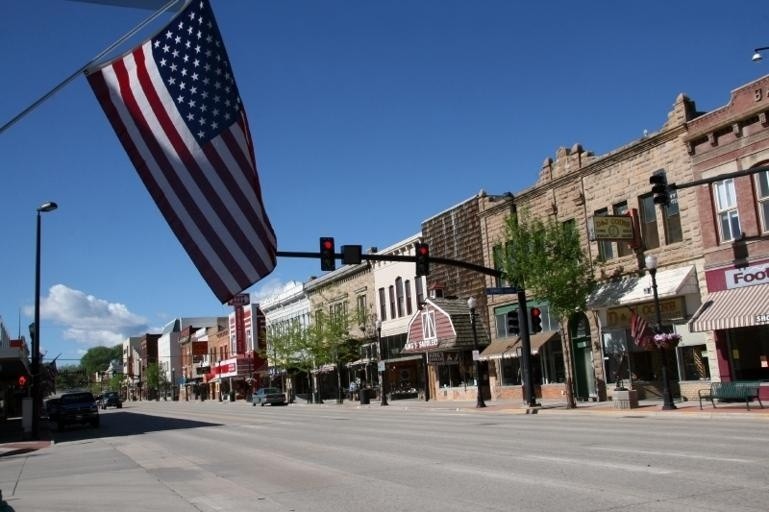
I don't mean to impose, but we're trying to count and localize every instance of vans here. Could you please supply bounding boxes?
[58,391,99,429]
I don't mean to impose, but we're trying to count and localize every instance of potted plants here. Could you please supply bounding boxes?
[604,338,639,410]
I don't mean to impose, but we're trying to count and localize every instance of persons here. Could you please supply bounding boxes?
[349,378,356,400]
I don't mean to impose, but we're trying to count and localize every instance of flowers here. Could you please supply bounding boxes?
[654,334,681,348]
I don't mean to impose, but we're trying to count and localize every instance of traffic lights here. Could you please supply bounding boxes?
[416,241,429,276]
[526,306,543,336]
[319,236,336,270]
[505,308,519,338]
[341,246,361,263]
[649,169,672,207]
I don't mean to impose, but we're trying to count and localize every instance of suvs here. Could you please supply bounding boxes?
[100,390,122,409]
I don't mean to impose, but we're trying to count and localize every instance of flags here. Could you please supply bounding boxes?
[632,307,651,349]
[84,0,281,306]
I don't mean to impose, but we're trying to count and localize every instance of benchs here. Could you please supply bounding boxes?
[697,380,765,411]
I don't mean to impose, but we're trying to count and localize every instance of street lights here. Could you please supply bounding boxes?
[374,318,388,405]
[641,253,679,411]
[127,350,277,402]
[752,45,769,63]
[31,201,61,437]
[467,297,487,408]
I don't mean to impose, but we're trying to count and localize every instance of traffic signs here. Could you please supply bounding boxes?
[484,287,518,295]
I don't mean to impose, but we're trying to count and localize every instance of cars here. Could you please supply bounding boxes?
[252,386,286,407]
